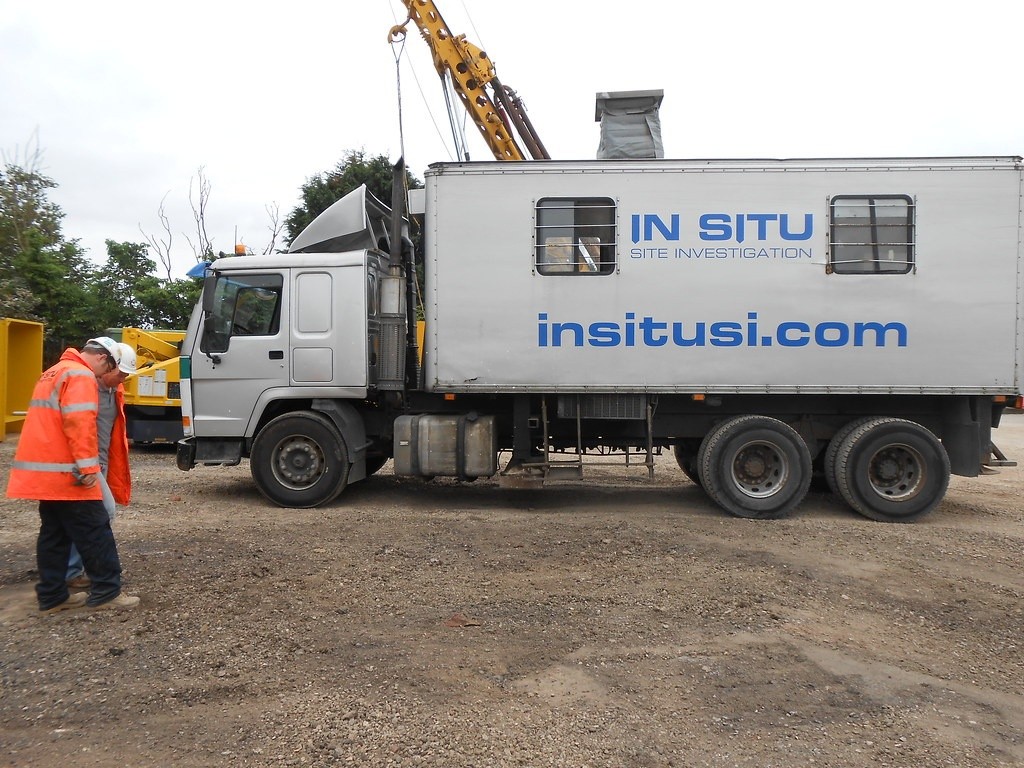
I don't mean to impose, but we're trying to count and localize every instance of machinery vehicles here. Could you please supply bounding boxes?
[387,0,603,371]
[1,314,188,450]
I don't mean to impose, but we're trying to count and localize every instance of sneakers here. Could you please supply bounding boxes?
[38,592,88,618]
[96,594,140,610]
[66,573,91,587]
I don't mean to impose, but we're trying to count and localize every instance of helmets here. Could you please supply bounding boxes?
[86,337,122,374]
[116,343,136,375]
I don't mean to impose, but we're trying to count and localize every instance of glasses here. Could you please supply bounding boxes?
[99,353,112,374]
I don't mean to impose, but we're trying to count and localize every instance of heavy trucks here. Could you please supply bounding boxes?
[171,150,1024,520]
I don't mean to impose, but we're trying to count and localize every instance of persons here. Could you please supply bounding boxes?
[5,336,140,615]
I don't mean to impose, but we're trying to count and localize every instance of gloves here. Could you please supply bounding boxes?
[72,464,86,486]
